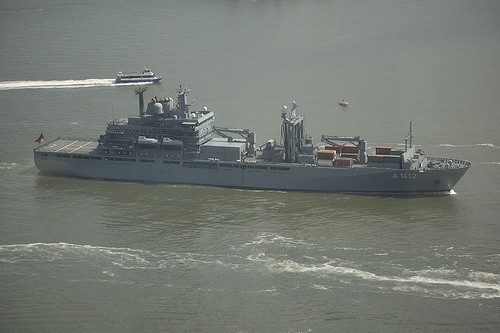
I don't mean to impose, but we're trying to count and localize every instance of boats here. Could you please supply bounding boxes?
[115,67,162,83]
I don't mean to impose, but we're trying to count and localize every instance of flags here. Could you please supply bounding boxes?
[34,133,45,146]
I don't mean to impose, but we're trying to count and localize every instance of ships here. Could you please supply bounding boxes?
[32,87,473,196]
